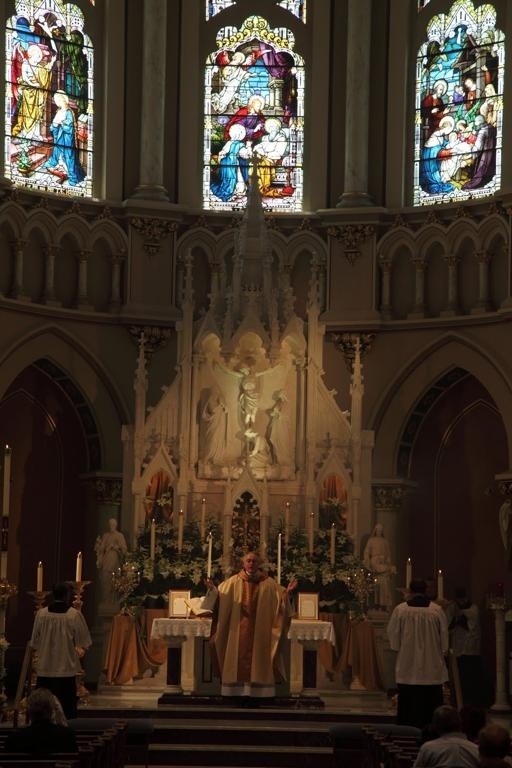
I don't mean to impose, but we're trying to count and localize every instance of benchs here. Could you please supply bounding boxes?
[0,720,134,768]
[330,726,512,768]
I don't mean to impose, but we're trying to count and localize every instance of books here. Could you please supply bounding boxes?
[186,597,215,616]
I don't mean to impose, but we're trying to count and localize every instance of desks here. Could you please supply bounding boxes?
[150,618,337,696]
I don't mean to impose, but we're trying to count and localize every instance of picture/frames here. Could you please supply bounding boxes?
[167,588,192,618]
[296,591,319,621]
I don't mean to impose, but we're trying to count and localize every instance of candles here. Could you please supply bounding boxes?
[308,511,317,554]
[437,570,444,599]
[285,501,290,541]
[276,532,281,586]
[405,557,412,591]
[330,519,337,563]
[207,531,212,579]
[111,561,141,589]
[76,550,83,581]
[177,508,185,554]
[199,499,206,538]
[150,519,156,555]
[37,559,44,593]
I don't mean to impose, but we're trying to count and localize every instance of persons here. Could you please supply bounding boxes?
[413,703,480,768]
[214,354,287,452]
[203,550,299,700]
[26,580,93,720]
[361,521,395,613]
[94,518,128,614]
[9,686,79,752]
[478,721,511,768]
[384,577,453,725]
